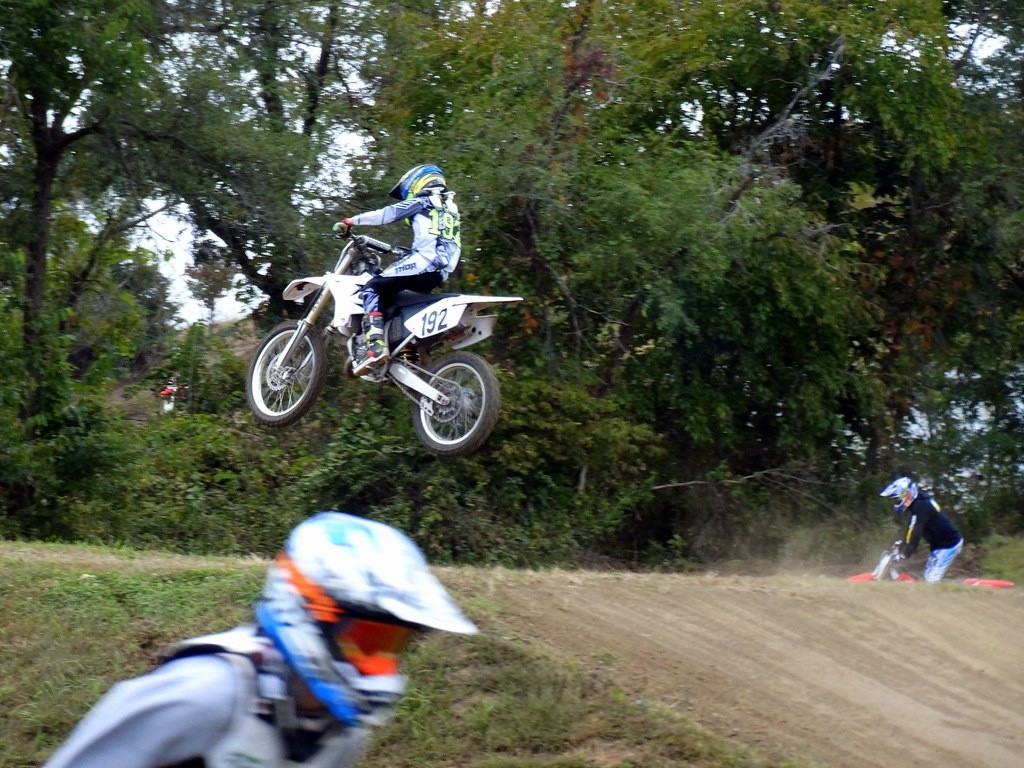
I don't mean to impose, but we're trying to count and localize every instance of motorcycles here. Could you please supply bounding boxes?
[848,539,1016,588]
[245,223,524,458]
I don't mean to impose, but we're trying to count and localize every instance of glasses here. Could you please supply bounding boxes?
[320,605,414,675]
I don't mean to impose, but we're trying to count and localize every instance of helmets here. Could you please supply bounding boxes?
[880,476,919,513]
[252,512,478,731]
[167,376,176,385]
[389,164,447,200]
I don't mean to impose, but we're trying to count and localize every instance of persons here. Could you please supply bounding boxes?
[44,511,477,768]
[163,377,178,412]
[881,476,964,584]
[333,163,461,377]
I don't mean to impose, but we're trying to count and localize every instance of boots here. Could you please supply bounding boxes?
[353,312,390,376]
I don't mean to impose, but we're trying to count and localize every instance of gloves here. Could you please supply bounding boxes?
[892,539,903,549]
[893,553,905,562]
[332,217,351,238]
